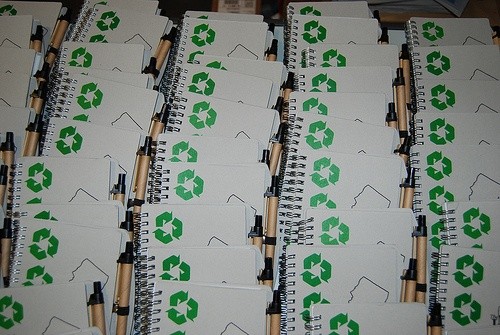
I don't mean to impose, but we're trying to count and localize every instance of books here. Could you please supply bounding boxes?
[0,0,500,335]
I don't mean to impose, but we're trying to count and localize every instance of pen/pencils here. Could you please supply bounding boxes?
[88,26,178,335]
[246,23,296,335]
[1,10,74,289]
[371,9,444,334]
